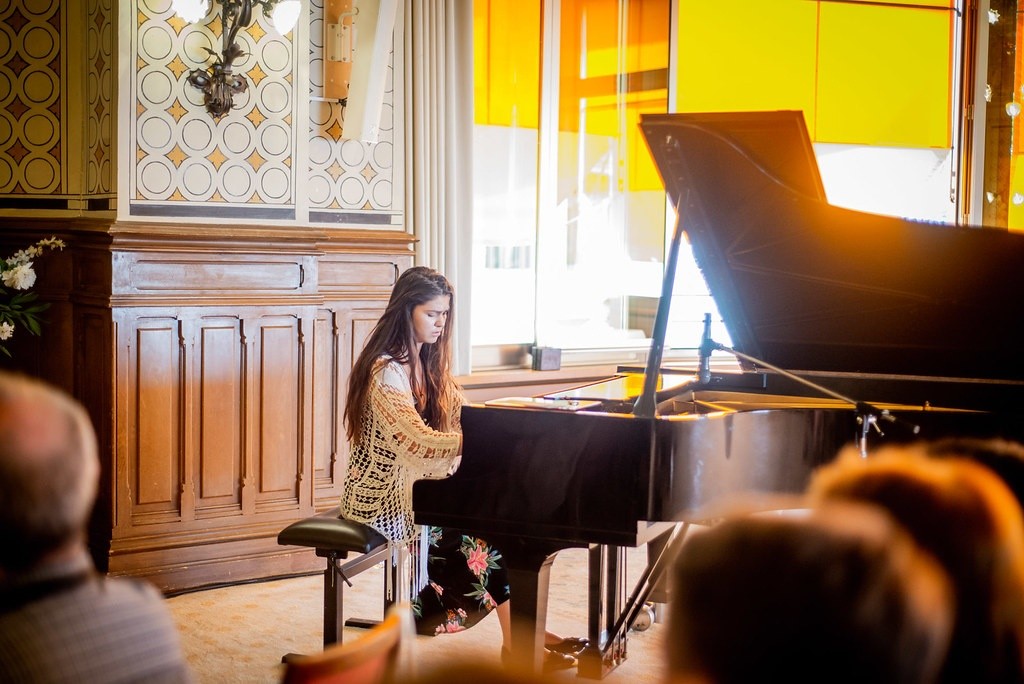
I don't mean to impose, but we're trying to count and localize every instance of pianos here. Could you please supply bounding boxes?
[411,107,1024,684]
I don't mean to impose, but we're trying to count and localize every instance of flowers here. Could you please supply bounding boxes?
[0,235,64,361]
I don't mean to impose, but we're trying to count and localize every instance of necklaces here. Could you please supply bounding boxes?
[403,354,426,404]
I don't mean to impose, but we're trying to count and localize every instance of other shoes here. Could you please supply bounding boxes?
[501,642,576,672]
[547,636,588,655]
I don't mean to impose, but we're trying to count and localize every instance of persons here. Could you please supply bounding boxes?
[0,371,187,684]
[338,265,591,675]
[656,442,1024,684]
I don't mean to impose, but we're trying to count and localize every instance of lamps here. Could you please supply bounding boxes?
[172,0,301,120]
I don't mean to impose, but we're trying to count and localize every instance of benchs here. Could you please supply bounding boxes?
[277,503,429,666]
